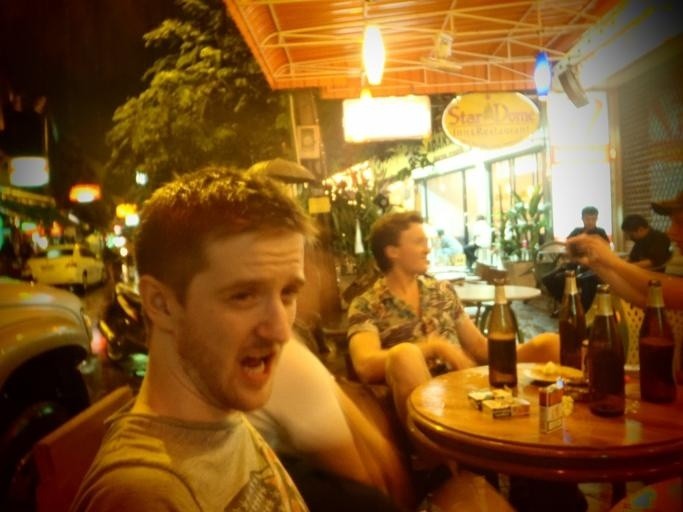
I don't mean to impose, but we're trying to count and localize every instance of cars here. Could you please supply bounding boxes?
[25,244,108,297]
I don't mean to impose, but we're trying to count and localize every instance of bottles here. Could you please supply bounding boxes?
[487,275,517,389]
[558,270,584,366]
[588,283,626,415]
[639,280,674,403]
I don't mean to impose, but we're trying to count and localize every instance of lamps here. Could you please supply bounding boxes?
[532,0,550,99]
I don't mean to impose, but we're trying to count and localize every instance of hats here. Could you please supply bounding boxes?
[649,191,682,216]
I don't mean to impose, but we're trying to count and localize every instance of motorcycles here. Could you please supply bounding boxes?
[97,282,149,366]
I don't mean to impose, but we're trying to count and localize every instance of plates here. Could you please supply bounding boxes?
[526,366,588,385]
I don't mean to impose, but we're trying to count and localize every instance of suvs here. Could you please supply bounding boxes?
[0,276,90,494]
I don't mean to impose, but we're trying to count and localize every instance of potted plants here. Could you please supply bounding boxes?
[498,184,553,286]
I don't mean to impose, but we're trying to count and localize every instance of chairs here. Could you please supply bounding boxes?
[10,383,133,512]
[308,311,361,384]
[535,241,568,279]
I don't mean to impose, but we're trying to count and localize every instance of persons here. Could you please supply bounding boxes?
[0,228,35,279]
[569,206,610,308]
[347,211,560,427]
[71,170,307,512]
[622,214,670,268]
[255,327,413,512]
[572,193,683,318]
